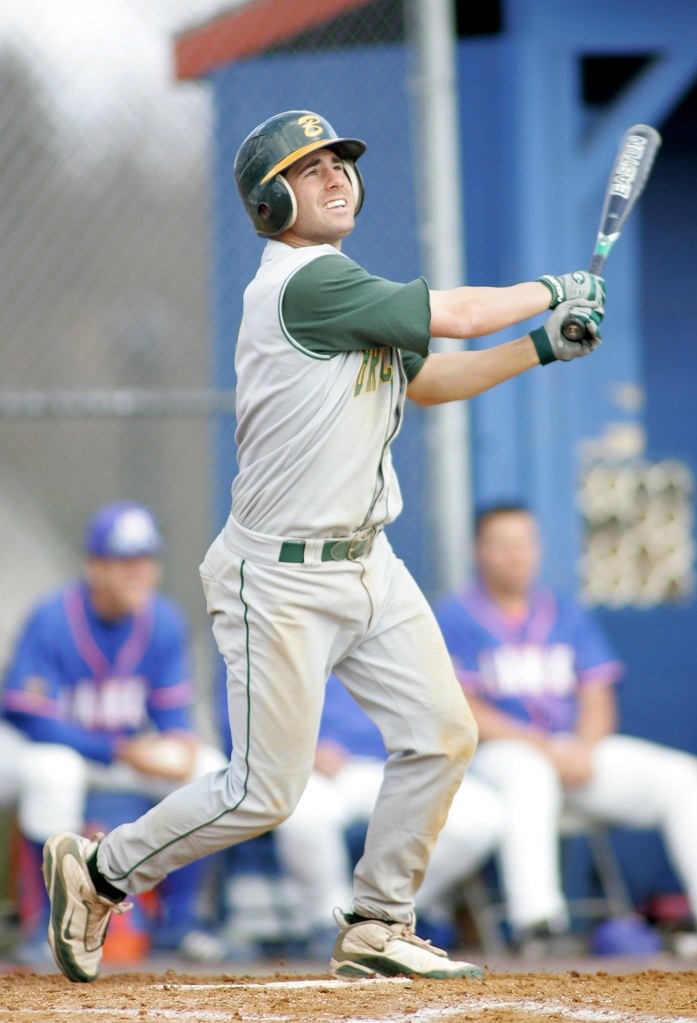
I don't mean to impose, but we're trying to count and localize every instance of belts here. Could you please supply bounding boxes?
[278,528,376,564]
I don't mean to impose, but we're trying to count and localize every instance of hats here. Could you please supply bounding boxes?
[84,504,162,558]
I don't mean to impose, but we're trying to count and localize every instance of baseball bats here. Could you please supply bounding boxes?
[559,121,664,342]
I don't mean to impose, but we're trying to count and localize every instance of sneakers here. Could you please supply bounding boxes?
[330,907,482,981]
[41,832,134,982]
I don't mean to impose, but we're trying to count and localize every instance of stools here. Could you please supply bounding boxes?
[456,810,629,960]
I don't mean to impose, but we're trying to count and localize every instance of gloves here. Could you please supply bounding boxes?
[527,298,605,366]
[535,271,606,311]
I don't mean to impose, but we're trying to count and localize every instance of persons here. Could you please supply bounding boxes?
[0,496,697,969]
[40,107,608,985]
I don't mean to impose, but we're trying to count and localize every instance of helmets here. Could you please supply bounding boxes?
[233,110,367,238]
